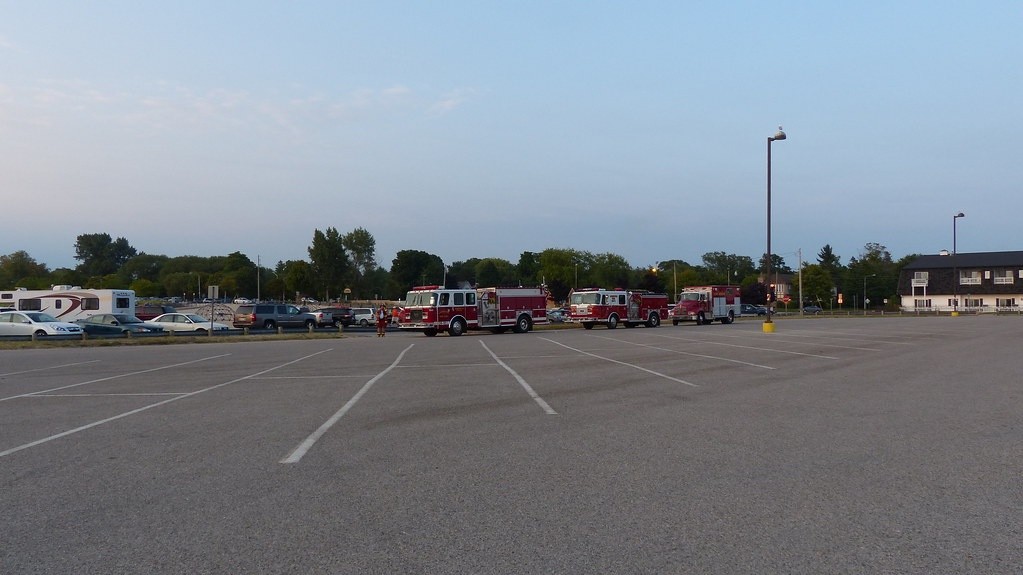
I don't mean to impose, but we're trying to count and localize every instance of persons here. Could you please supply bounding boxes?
[375,304,388,337]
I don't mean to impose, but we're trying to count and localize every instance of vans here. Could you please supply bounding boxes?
[233,304,317,331]
[313,307,356,329]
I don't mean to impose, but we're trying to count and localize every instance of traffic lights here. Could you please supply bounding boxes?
[769,283,776,293]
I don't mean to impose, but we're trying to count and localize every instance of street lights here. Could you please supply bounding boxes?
[727,259,739,286]
[761,125,788,332]
[864,273,877,316]
[951,213,964,316]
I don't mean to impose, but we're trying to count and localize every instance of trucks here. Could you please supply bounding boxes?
[13,285,136,324]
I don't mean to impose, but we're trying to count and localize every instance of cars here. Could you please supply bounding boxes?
[144,312,229,336]
[75,314,166,340]
[203,298,219,303]
[305,297,318,304]
[133,295,201,305]
[546,309,576,324]
[740,303,767,318]
[234,297,253,305]
[802,305,822,316]
[0,311,83,342]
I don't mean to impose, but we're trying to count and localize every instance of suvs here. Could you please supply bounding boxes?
[381,306,401,326]
[349,307,380,328]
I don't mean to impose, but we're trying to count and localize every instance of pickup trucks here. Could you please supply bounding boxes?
[288,306,333,329]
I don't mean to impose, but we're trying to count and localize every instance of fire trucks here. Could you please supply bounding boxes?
[568,287,669,330]
[398,286,547,334]
[670,286,741,326]
